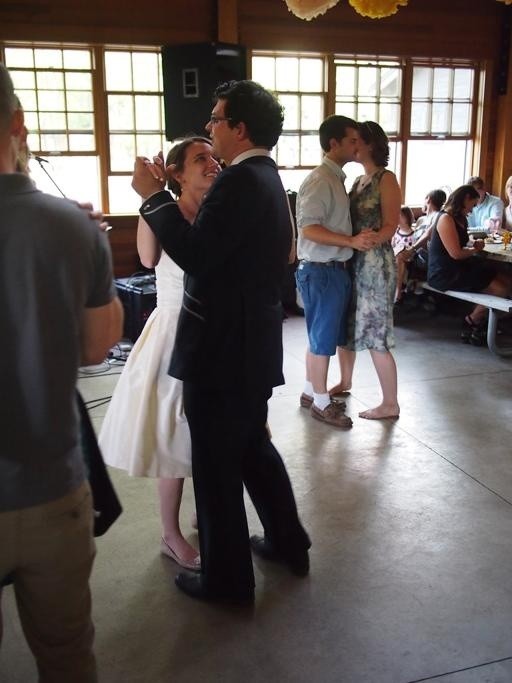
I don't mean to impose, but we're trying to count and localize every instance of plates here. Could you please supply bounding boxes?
[478,238,502,244]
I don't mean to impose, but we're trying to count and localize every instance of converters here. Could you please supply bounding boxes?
[120,344,132,351]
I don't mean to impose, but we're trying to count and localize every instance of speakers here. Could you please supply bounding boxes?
[162,39,253,142]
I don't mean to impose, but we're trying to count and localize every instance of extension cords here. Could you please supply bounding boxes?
[129,274,156,285]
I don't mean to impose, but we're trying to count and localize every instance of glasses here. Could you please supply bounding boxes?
[210,115,239,127]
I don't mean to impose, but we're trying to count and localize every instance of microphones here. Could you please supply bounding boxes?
[27,151,49,162]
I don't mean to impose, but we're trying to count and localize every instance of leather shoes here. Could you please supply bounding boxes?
[160,536,201,571]
[249,534,309,576]
[176,572,255,603]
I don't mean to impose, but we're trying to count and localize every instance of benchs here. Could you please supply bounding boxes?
[399,273,512,358]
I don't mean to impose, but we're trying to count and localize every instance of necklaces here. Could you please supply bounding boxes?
[360,168,378,187]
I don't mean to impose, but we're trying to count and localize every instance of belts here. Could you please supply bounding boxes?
[300,259,350,270]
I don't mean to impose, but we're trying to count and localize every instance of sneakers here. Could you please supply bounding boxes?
[310,402,353,427]
[301,393,346,410]
[461,328,487,345]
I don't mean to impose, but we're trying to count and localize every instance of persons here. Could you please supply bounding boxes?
[425,184,509,349]
[392,205,416,259]
[129,81,313,615]
[331,122,402,419]
[502,175,512,232]
[0,79,122,683]
[294,114,386,429]
[16,126,126,541]
[467,175,506,233]
[95,124,222,576]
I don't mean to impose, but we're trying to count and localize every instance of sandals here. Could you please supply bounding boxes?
[461,315,488,333]
